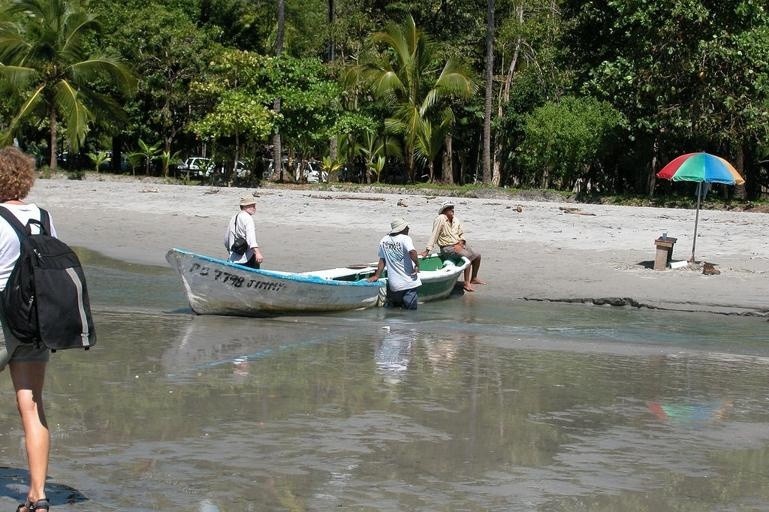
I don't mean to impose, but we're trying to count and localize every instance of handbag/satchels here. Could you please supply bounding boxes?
[232,211,247,255]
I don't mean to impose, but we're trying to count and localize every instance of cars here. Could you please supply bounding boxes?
[262,159,274,179]
[200,160,251,178]
[282,159,328,183]
[177,158,216,176]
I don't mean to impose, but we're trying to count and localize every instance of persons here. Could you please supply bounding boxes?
[367,219,418,310]
[0,145,58,512]
[224,194,262,269]
[418,202,487,292]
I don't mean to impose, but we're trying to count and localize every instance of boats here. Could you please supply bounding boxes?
[161,317,363,374]
[166,247,471,315]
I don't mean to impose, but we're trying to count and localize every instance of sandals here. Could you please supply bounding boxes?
[16,498,50,512]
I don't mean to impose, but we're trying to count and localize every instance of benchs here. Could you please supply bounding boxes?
[654,236,676,270]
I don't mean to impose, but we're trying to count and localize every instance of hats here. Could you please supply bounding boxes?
[388,219,408,233]
[438,200,455,214]
[239,196,257,206]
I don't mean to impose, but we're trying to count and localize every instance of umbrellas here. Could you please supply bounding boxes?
[657,151,745,263]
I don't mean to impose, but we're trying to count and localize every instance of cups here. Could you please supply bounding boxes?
[663,232,667,241]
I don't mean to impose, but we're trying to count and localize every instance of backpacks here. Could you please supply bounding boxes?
[0,203,97,353]
